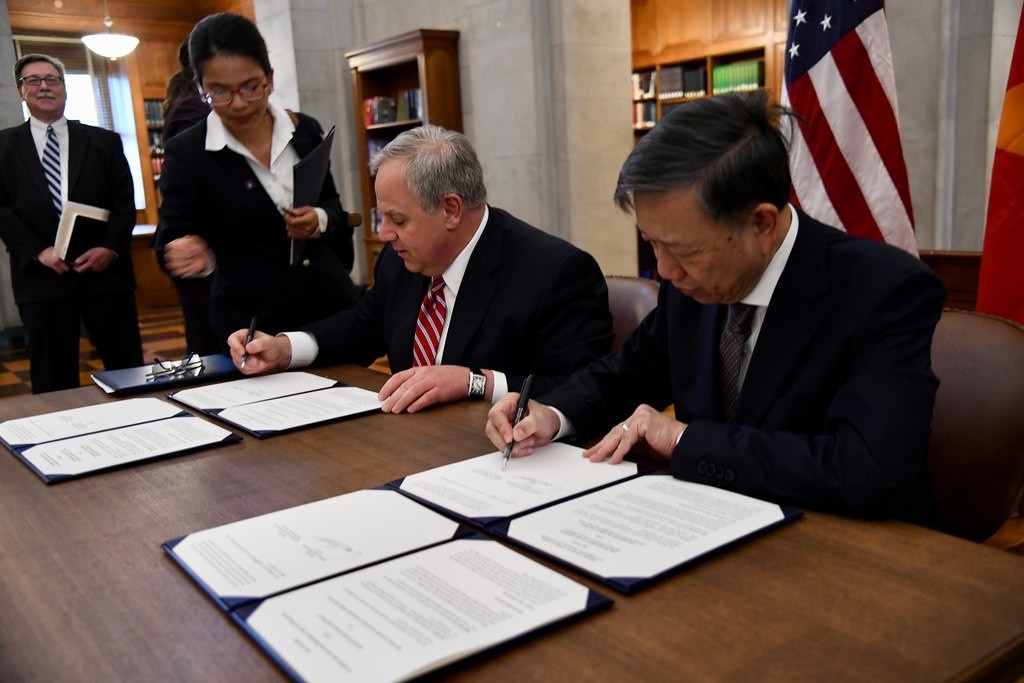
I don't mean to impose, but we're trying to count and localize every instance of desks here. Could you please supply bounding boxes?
[0,365,1024,683]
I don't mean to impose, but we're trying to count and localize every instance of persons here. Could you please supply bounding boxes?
[156,11,354,365]
[227,124,610,416]
[0,53,138,393]
[485,93,944,518]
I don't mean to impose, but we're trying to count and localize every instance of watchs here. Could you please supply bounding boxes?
[466,366,486,399]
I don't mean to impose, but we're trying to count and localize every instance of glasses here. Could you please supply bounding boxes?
[196,73,272,107]
[146,351,204,377]
[19,74,62,86]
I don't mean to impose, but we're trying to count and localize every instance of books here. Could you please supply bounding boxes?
[365,89,422,126]
[368,140,387,165]
[631,61,763,129]
[371,206,384,233]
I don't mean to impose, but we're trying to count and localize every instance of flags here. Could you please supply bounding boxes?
[976,0,1024,331]
[777,0,921,258]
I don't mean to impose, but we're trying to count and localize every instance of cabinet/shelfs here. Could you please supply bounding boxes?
[630,0,790,146]
[344,28,463,287]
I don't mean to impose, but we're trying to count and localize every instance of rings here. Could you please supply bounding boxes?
[622,424,629,431]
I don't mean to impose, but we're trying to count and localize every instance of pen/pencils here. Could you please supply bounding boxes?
[502,372,536,470]
[240,317,254,369]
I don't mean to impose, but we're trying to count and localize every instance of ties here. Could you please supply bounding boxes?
[721,302,757,422]
[413,276,447,369]
[41,125,62,216]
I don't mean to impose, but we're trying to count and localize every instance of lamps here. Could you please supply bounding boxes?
[82,0,139,61]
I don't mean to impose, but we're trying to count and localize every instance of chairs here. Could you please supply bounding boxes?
[930,309,1024,544]
[604,274,660,353]
